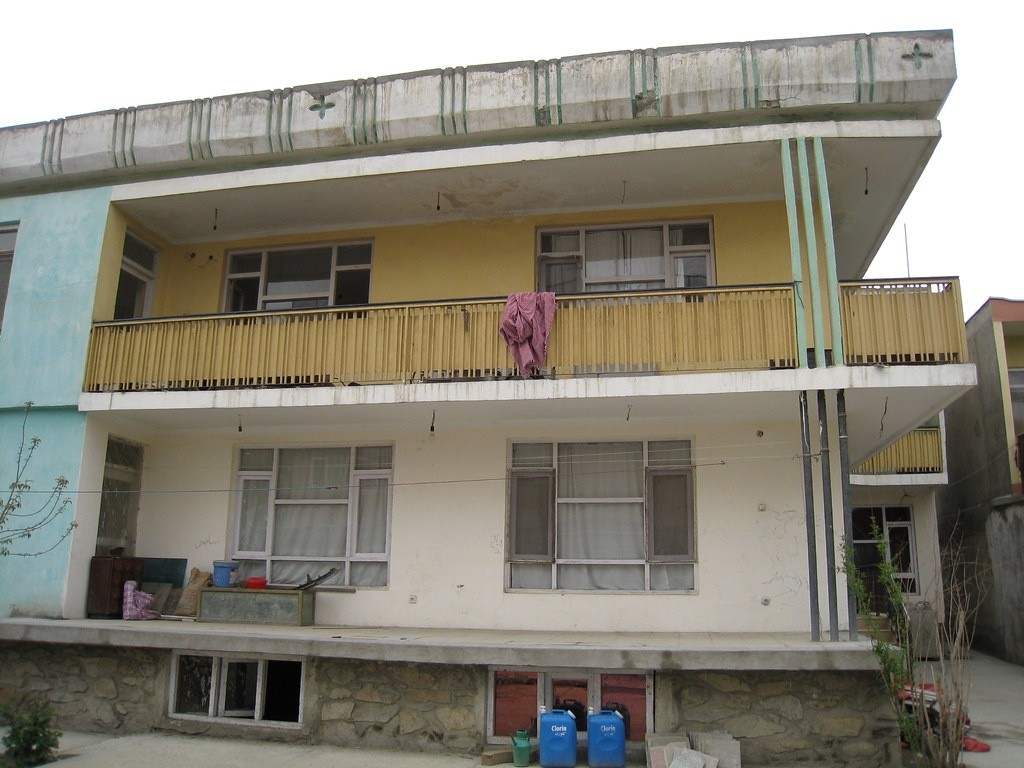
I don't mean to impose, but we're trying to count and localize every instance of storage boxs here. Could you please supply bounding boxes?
[196,587,317,627]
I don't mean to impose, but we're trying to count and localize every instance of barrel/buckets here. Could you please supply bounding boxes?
[213,559,240,588]
[246,577,268,590]
[587,706,626,767]
[539,705,578,766]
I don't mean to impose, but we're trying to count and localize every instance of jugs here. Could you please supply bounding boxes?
[509,729,532,767]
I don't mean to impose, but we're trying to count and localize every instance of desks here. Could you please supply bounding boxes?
[88,556,187,621]
